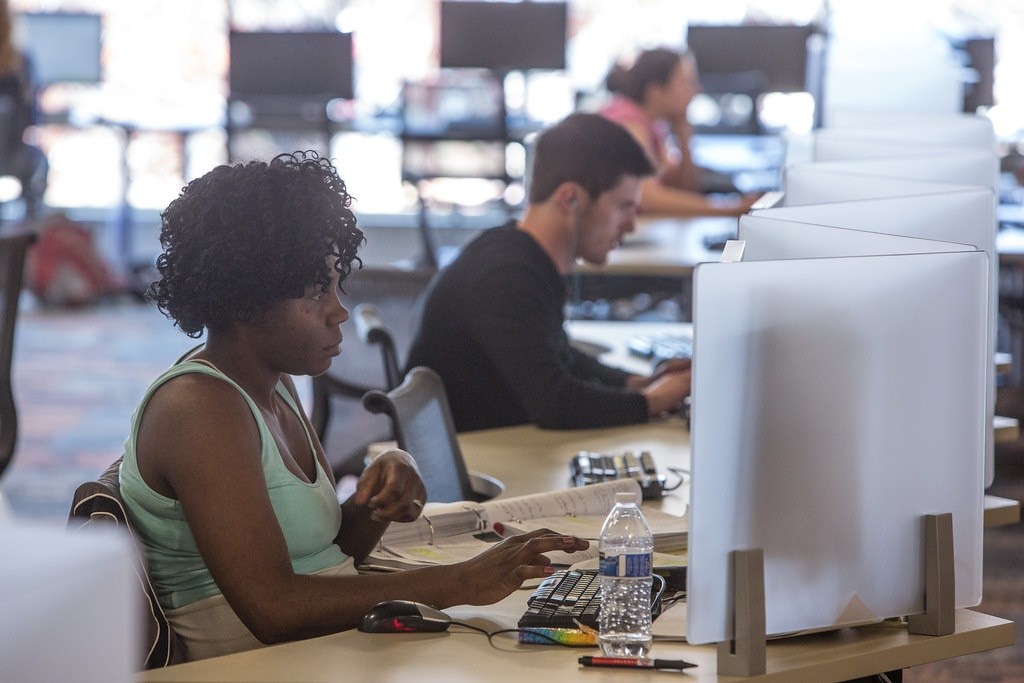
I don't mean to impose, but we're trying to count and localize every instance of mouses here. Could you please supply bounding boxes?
[357,602,451,633]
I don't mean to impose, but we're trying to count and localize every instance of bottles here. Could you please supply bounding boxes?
[599,493,653,658]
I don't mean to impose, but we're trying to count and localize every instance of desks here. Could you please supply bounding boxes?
[138,212,1024,683]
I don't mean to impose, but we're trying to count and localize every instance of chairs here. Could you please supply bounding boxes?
[307,374,392,480]
[356,302,406,389]
[400,72,530,258]
[66,456,182,671]
[362,365,478,505]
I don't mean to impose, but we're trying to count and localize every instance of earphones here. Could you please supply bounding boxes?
[564,197,579,209]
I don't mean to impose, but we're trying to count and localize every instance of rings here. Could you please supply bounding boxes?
[413,499,423,510]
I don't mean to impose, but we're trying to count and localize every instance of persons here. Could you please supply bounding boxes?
[0,1,47,474]
[118,151,590,659]
[403,114,691,433]
[596,50,765,216]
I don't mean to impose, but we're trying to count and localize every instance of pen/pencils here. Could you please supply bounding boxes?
[578,656,699,670]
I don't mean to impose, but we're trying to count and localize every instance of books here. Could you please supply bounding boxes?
[361,479,688,573]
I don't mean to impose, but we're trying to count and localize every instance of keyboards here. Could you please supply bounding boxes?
[628,331,694,361]
[568,450,661,502]
[518,568,607,632]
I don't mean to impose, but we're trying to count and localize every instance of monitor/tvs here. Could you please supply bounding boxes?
[681,115,1001,645]
